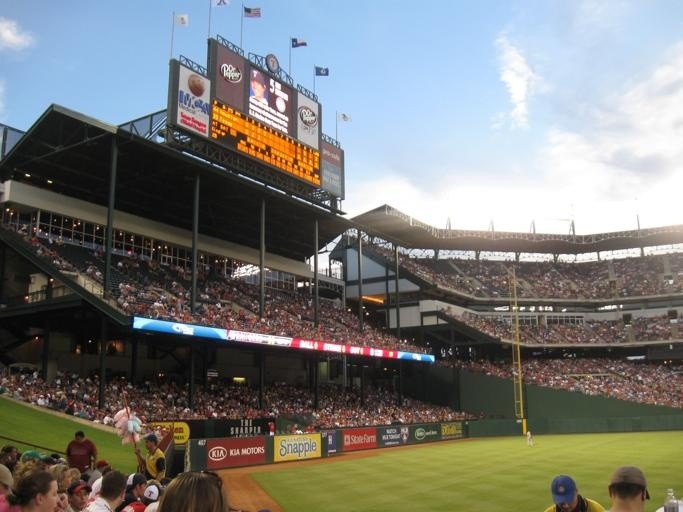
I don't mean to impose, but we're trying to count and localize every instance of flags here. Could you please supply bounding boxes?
[314,66,328,76]
[335,112,353,122]
[243,6,261,18]
[214,0,229,7]
[174,14,188,27]
[290,37,307,48]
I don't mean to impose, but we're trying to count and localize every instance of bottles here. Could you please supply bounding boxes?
[664,488,679,512]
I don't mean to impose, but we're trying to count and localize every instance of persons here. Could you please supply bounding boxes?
[604,465,650,511]
[0,432,234,512]
[538,474,606,512]
[0,232,682,431]
[525,430,534,448]
[247,67,269,105]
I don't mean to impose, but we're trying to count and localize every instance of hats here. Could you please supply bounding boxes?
[143,484,159,504]
[143,433,157,441]
[608,466,650,501]
[552,476,576,504]
[126,473,147,491]
[67,480,92,495]
